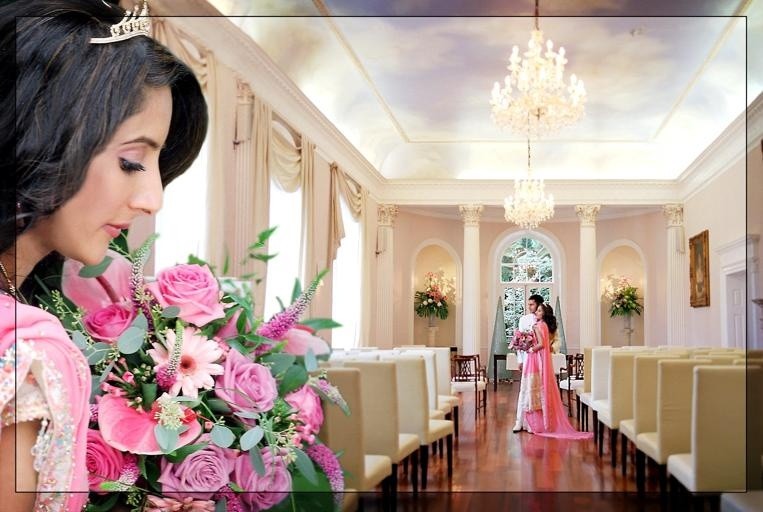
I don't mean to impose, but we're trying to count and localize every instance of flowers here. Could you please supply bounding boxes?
[414,271,450,322]
[27,206,357,512]
[507,329,534,353]
[601,279,645,319]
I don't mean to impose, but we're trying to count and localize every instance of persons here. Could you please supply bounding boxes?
[0,0,209,512]
[694,251,704,300]
[524,302,594,440]
[511,295,561,434]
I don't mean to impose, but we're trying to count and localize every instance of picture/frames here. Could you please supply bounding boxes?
[688,230,710,308]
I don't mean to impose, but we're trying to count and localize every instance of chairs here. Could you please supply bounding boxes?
[300,341,492,512]
[555,342,763,512]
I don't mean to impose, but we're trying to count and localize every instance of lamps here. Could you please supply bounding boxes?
[488,0,590,138]
[503,127,557,231]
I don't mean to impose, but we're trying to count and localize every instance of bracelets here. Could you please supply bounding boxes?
[528,347,534,353]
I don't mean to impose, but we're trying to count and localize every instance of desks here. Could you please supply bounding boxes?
[493,352,573,393]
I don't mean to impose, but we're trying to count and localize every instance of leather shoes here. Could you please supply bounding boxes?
[513,426,523,433]
